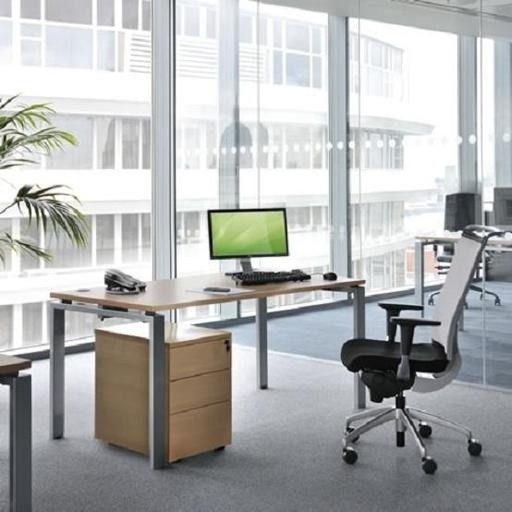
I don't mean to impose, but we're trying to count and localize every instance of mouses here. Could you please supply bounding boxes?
[324,271,338,282]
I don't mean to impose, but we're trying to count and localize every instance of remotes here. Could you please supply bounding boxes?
[203,286,230,293]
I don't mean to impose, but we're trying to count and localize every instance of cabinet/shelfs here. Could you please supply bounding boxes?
[95,332,232,463]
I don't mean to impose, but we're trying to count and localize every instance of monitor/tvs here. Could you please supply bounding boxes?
[206,207,289,260]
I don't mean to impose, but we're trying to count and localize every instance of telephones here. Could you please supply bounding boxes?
[105,269,146,291]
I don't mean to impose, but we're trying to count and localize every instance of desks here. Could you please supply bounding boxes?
[46,269,367,470]
[413,228,512,332]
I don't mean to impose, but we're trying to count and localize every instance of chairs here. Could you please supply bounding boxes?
[340,222,505,476]
[428,192,501,310]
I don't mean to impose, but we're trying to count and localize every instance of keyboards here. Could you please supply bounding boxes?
[224,269,312,286]
